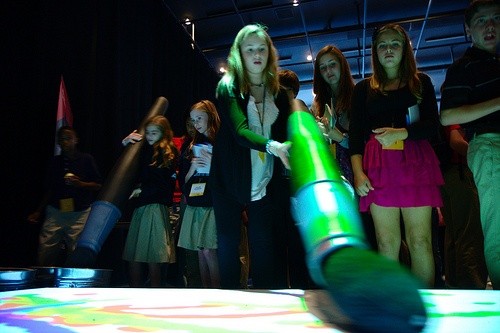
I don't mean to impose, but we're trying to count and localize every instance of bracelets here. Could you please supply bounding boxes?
[266,140,273,155]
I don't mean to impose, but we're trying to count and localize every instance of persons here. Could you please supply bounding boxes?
[121,0,500,289]
[28,126,103,268]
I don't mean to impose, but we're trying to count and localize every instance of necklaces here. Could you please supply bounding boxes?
[245,77,264,88]
[383,78,403,90]
[248,86,266,162]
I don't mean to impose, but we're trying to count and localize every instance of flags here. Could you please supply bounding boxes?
[54,76,75,155]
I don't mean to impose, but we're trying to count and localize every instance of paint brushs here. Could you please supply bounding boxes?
[281,99,428,333]
[65,96,169,268]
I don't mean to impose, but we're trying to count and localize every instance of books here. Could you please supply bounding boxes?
[406,114,410,127]
[409,104,420,126]
[323,103,333,144]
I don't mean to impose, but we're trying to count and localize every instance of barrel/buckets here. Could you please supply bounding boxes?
[0,267,38,292]
[54,268,111,288]
